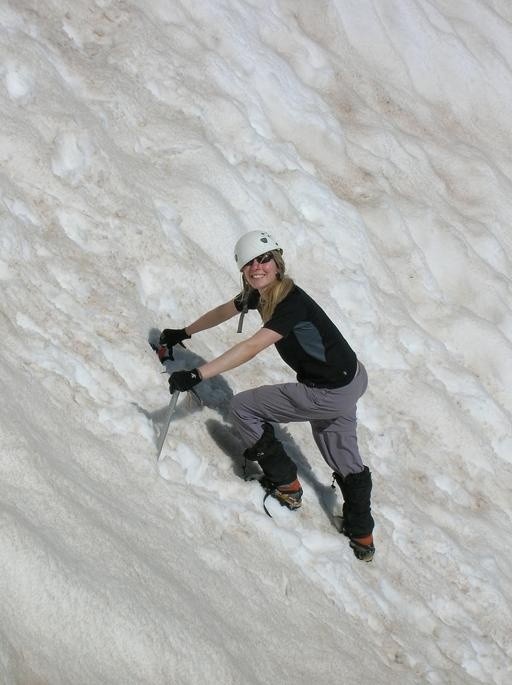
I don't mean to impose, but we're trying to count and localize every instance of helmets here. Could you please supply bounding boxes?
[234,231,283,272]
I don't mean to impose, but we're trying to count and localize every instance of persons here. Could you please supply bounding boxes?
[158,229,376,563]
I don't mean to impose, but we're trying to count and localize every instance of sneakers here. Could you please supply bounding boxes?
[244,473,303,510]
[339,517,375,561]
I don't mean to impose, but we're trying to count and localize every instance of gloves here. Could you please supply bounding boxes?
[159,328,191,349]
[168,368,201,393]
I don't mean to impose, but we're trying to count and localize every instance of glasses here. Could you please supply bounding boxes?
[245,253,271,266]
